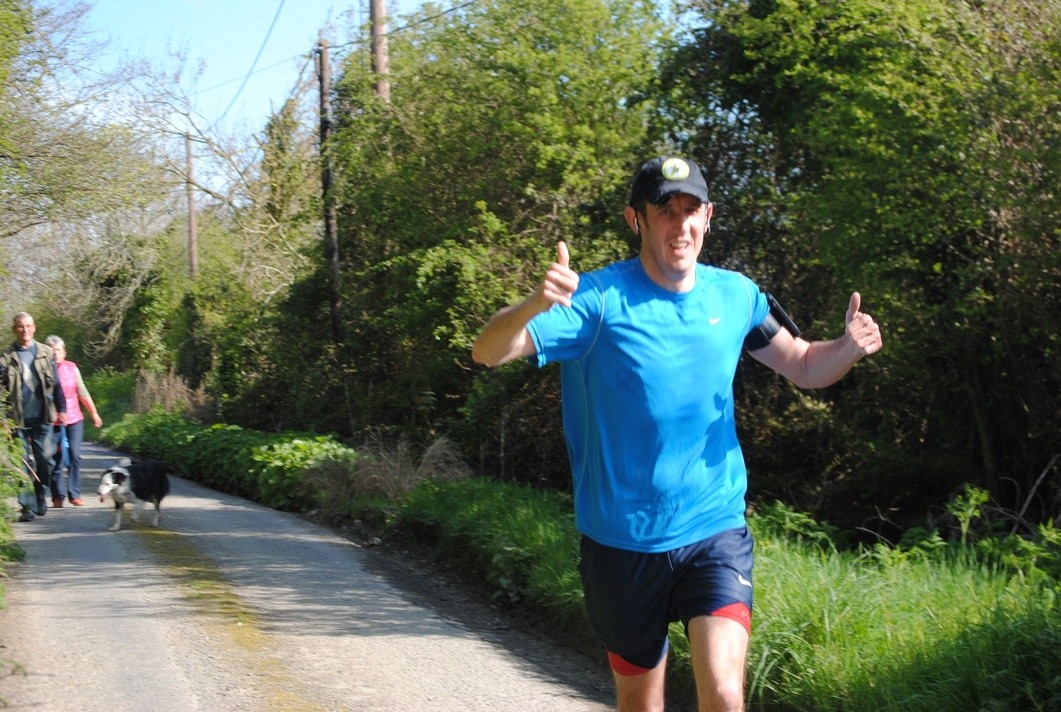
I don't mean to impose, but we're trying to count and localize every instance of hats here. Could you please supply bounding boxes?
[632,156,709,205]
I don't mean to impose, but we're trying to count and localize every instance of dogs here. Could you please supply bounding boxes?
[96,458,169,531]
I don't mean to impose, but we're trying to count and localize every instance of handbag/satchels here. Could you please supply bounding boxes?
[52,422,69,467]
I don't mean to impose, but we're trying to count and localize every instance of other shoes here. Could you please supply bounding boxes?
[37,499,46,516]
[54,499,62,507]
[19,512,34,521]
[69,499,83,506]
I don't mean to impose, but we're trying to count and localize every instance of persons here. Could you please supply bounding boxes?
[470,154,882,711]
[0,312,103,522]
